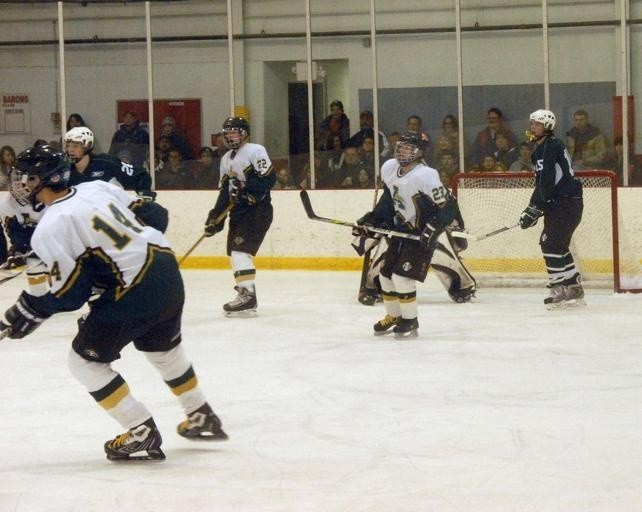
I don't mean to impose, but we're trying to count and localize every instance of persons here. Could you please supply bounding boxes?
[405,115,432,165]
[67,113,85,129]
[196,147,220,186]
[360,137,385,165]
[439,169,452,187]
[604,136,642,187]
[434,114,470,155]
[164,149,195,185]
[343,143,363,174]
[0,143,228,461]
[305,150,322,166]
[159,115,191,159]
[521,110,585,311]
[352,165,373,188]
[349,109,389,156]
[142,148,164,177]
[158,135,179,161]
[323,132,346,179]
[277,167,295,190]
[213,131,229,157]
[436,150,461,169]
[0,145,48,272]
[62,128,157,202]
[566,110,607,168]
[116,149,134,164]
[203,117,276,319]
[468,153,505,173]
[510,142,536,172]
[494,131,519,169]
[296,164,324,189]
[108,110,148,167]
[351,128,451,339]
[317,100,352,150]
[468,107,517,163]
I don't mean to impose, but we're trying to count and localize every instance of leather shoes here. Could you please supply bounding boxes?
[0,296,47,341]
[351,214,376,236]
[205,207,225,236]
[518,202,543,227]
[233,179,267,205]
[420,216,444,251]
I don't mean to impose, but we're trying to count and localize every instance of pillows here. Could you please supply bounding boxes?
[528,109,555,131]
[224,118,250,148]
[65,127,95,158]
[9,144,69,211]
[397,131,431,168]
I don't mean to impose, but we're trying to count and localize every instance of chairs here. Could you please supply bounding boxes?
[105,421,160,453]
[394,320,419,332]
[178,405,221,434]
[223,296,257,312]
[565,286,584,300]
[373,316,394,331]
[544,294,565,302]
